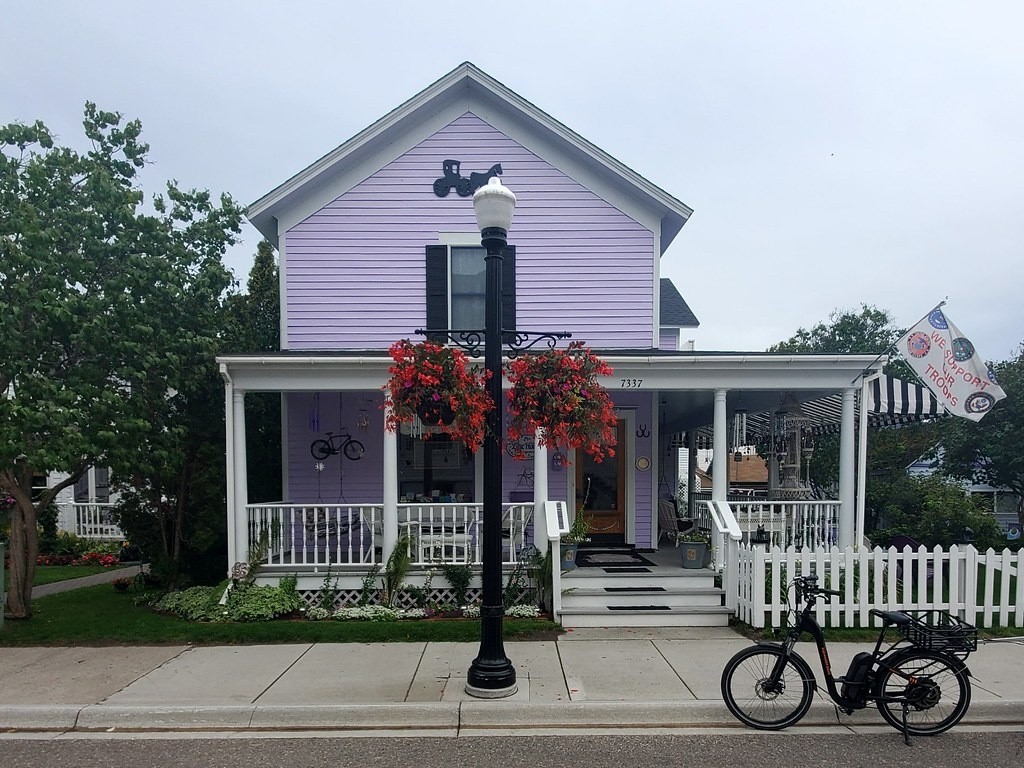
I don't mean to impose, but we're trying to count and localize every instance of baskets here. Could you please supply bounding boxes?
[898,609,978,654]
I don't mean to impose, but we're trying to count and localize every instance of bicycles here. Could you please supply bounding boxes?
[720,573,982,747]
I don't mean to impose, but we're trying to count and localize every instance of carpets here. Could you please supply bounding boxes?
[575,550,658,568]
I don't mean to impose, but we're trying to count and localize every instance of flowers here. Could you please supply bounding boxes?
[509,342,616,461]
[382,340,495,453]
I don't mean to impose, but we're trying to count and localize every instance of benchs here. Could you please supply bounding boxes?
[398,506,474,533]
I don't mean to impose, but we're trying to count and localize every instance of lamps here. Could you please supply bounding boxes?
[734,414,744,462]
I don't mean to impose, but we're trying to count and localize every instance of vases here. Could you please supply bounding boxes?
[410,392,457,425]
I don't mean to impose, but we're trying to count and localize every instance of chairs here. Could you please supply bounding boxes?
[854,531,949,591]
[658,499,694,548]
[502,506,533,570]
[359,508,419,562]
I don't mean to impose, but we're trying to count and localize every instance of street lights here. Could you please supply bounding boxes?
[460,175,521,704]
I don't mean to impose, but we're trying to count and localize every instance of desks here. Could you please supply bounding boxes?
[411,534,473,562]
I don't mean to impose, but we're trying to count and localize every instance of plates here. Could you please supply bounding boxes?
[635,456,651,471]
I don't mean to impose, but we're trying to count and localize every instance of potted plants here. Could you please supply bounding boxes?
[678,533,708,569]
[560,477,593,570]
